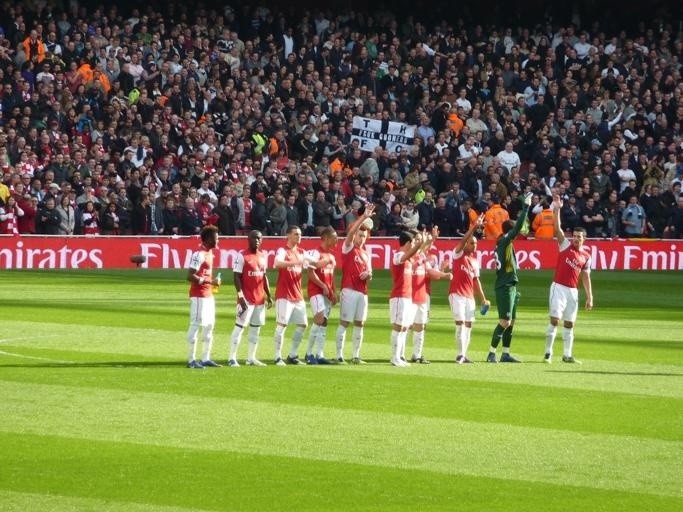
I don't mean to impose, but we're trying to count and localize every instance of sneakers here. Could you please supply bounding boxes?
[185,354,366,370]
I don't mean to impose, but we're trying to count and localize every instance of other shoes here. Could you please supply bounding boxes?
[456,355,474,364]
[562,356,582,365]
[486,351,499,362]
[390,356,430,367]
[500,354,521,362]
[543,354,553,363]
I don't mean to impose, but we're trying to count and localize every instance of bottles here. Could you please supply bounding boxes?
[480,300,490,316]
[211,272,221,294]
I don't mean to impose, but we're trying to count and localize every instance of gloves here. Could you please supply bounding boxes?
[524,191,534,207]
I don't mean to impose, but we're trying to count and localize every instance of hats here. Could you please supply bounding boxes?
[48,183,62,191]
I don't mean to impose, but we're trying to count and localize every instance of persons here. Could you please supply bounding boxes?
[227,230,272,367]
[539,195,594,366]
[271,223,310,367]
[305,224,339,367]
[335,200,378,365]
[486,190,536,363]
[185,222,223,369]
[386,225,453,369]
[445,212,492,366]
[2,1,682,240]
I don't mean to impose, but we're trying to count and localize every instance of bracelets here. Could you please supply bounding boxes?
[237,290,244,299]
[198,277,205,285]
